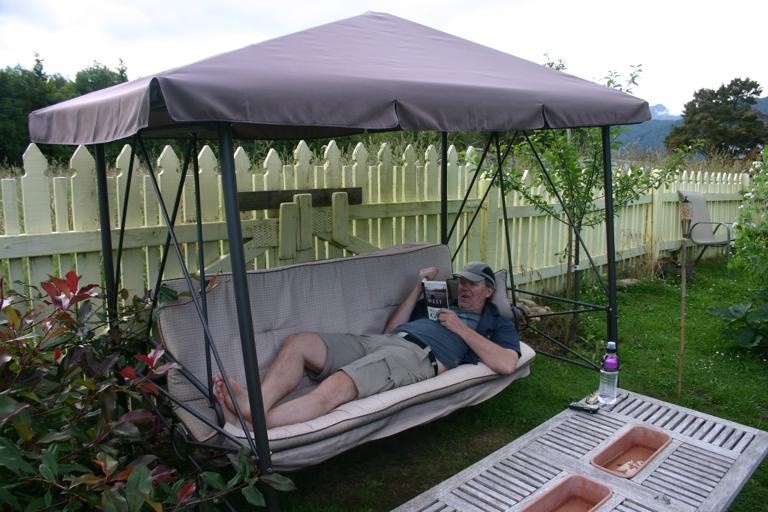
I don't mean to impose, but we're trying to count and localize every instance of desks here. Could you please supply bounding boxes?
[387,385,766,510]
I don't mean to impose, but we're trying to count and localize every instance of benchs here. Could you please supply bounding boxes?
[152,242,538,468]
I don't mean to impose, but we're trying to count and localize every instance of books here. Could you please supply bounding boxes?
[420,275,450,323]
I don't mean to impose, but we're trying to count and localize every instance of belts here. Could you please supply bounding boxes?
[393,330,439,376]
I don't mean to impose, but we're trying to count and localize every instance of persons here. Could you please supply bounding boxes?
[212,259,522,432]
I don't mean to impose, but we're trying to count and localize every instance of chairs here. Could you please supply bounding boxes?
[676,190,740,262]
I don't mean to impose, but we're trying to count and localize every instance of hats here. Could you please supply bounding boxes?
[451,259,497,289]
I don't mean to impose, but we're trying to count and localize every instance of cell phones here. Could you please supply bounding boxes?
[569,401,599,414]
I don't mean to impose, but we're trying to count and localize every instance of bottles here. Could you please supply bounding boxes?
[596,340,620,406]
